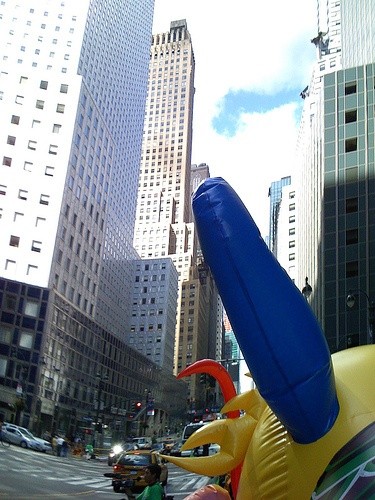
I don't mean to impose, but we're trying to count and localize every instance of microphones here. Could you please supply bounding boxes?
[104,473,121,479]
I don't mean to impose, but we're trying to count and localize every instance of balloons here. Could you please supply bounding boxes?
[159,177,375,500]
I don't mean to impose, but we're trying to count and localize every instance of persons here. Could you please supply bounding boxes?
[193,446,200,456]
[50,434,68,456]
[153,420,187,437]
[202,443,211,456]
[120,463,166,500]
[76,437,96,459]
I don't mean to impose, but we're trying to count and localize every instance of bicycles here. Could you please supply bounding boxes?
[0,430,10,448]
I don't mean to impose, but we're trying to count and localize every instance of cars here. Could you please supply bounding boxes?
[108,437,182,466]
[0,422,53,452]
[113,448,168,493]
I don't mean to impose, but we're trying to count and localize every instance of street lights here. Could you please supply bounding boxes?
[346,288,375,345]
[90,373,109,460]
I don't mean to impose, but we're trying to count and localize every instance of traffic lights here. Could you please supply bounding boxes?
[200,373,206,384]
[209,376,216,387]
[154,409,158,414]
[136,401,141,409]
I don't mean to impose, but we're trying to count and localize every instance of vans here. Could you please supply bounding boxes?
[180,420,220,457]
